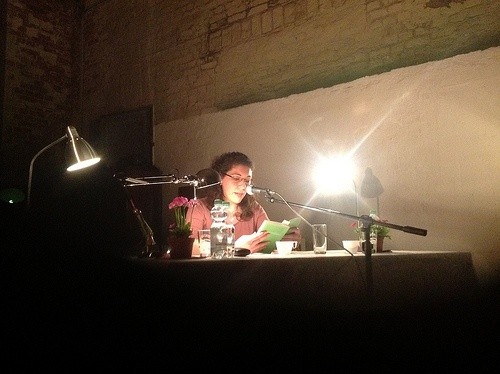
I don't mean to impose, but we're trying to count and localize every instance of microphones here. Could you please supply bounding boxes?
[246,185,276,196]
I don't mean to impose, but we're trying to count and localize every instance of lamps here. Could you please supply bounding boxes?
[25,125,102,208]
[114,168,222,260]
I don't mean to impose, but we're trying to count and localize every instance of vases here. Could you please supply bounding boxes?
[370,237,384,251]
[168,238,195,258]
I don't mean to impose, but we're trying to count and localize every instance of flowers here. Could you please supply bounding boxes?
[352,211,392,239]
[168,196,192,237]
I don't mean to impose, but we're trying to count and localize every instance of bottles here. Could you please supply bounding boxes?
[210,199,235,258]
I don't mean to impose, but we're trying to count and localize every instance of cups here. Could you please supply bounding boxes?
[276,240,297,256]
[342,240,359,254]
[312,224,328,253]
[197,229,211,258]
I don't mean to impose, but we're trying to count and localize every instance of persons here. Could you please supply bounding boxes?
[184,152,301,258]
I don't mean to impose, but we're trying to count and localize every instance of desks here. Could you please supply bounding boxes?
[124,250,478,374]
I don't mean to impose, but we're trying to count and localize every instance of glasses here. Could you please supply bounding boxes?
[223,172,252,184]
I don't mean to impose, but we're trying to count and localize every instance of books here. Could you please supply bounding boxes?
[257,217,301,254]
